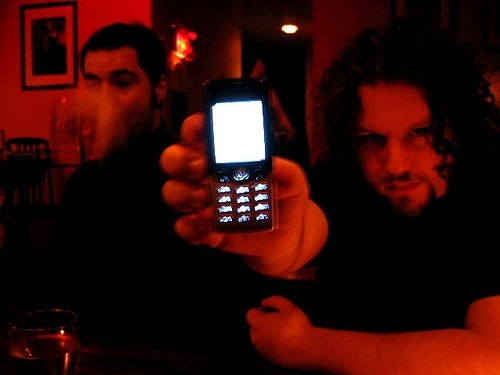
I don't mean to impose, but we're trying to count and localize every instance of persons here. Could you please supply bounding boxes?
[42,22,173,351]
[155,22,499,375]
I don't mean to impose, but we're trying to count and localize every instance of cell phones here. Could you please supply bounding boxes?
[203,79,277,233]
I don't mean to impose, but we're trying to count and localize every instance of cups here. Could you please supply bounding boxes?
[7,309,81,375]
